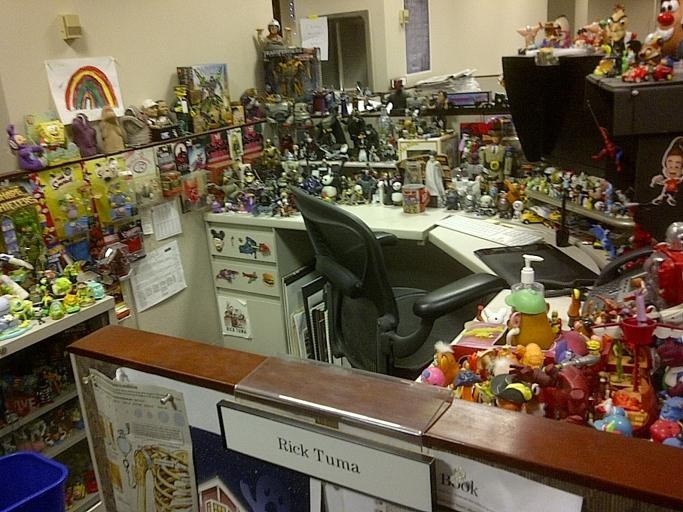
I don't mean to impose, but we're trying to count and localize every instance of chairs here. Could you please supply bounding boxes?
[287,185,505,379]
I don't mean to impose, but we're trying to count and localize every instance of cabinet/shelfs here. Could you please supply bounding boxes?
[0,293,168,512]
[586,74,683,238]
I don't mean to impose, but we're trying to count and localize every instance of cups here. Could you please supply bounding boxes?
[402,184,432,215]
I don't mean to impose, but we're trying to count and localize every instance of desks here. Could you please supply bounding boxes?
[204,175,458,360]
[416,209,598,386]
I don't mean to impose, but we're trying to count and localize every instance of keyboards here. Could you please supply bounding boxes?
[432,214,542,247]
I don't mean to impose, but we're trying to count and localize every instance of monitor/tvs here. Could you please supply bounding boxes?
[501,57,608,178]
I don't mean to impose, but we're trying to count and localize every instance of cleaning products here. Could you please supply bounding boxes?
[505,255,553,350]
